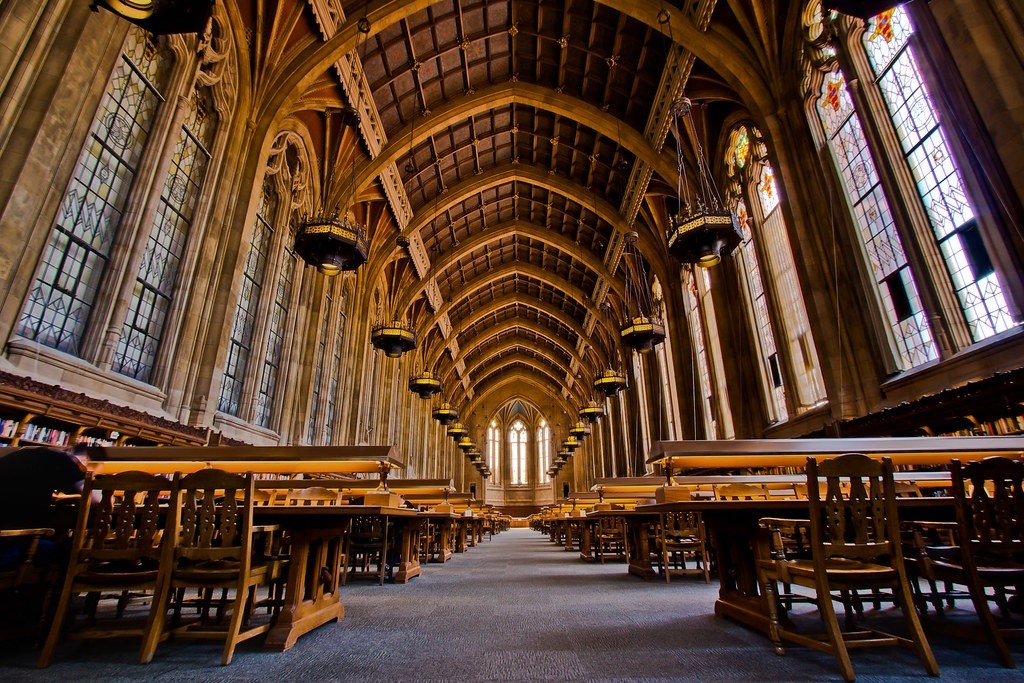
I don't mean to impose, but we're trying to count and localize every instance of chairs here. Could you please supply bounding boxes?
[923,455,1024,669]
[752,454,941,681]
[529,454,966,614]
[0,468,436,664]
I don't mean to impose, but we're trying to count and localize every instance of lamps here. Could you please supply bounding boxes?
[291,0,370,277]
[446,277,493,479]
[372,64,429,358]
[546,404,591,478]
[432,300,459,426]
[594,303,629,398]
[656,9,744,268]
[578,345,604,424]
[409,163,443,399]
[619,231,665,353]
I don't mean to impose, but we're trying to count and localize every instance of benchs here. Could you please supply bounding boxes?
[530,434,1024,578]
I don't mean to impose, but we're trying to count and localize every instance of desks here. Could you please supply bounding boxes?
[647,435,1024,648]
[50,445,416,652]
[448,493,513,554]
[248,479,461,584]
